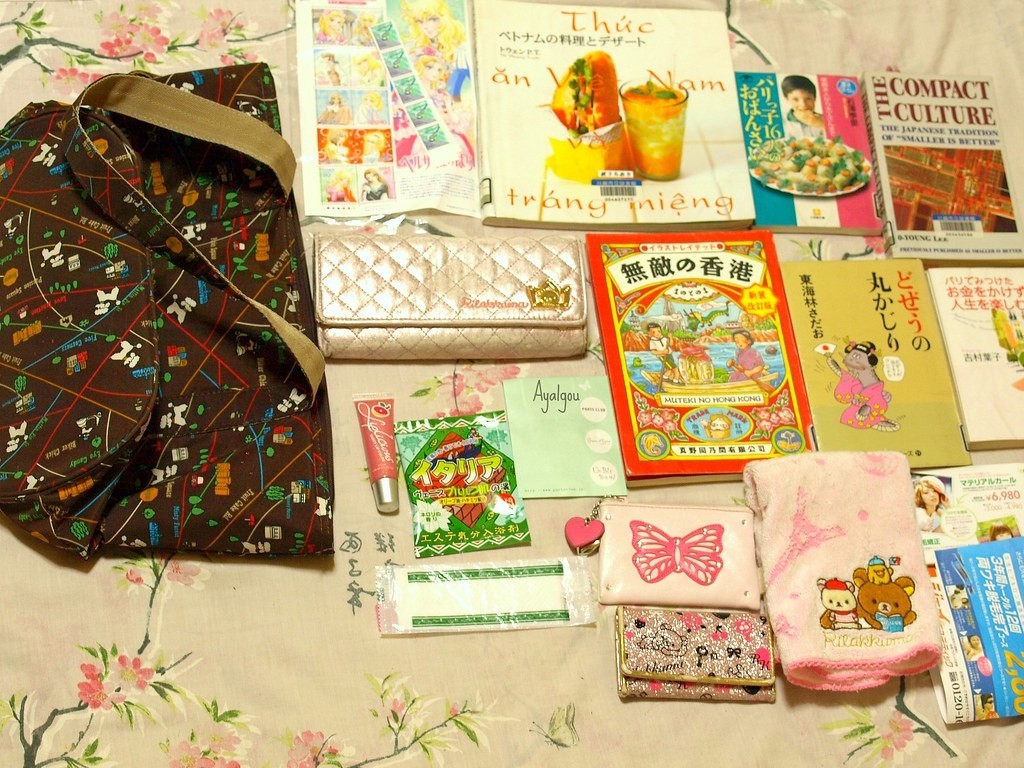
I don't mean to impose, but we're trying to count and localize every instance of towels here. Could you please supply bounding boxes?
[739,449,946,694]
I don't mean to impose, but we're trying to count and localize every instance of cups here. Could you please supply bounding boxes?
[619,83,688,181]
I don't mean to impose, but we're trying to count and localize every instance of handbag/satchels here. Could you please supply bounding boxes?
[1,60,335,559]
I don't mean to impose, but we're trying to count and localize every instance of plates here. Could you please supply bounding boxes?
[749,135,871,200]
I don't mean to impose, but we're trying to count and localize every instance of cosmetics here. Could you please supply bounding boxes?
[352,391,401,513]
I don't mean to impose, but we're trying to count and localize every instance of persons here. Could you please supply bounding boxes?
[781,75,826,140]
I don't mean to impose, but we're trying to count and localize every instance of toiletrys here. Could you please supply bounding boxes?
[351,393,405,517]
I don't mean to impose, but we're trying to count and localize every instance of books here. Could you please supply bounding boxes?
[925,269,1024,451]
[860,74,1023,267]
[781,259,972,470]
[472,0,755,234]
[734,71,884,238]
[583,231,820,489]
